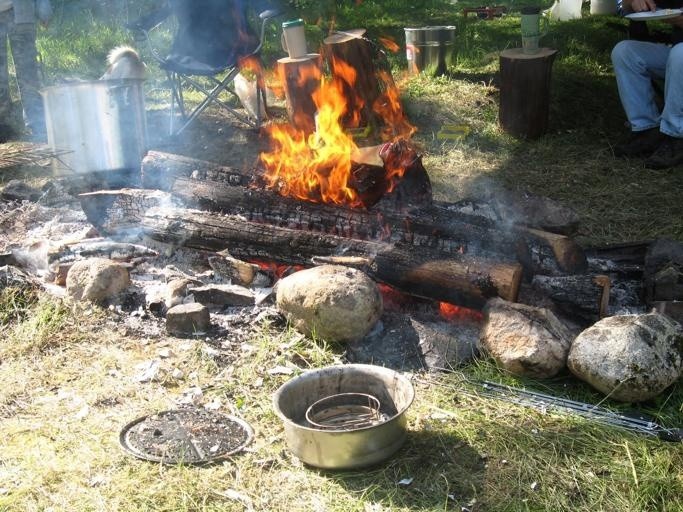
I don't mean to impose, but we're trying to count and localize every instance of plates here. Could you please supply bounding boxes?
[623,8,683,23]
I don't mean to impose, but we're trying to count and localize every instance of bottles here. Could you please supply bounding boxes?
[520,6,542,55]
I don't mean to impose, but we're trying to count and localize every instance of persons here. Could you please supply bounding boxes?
[611,0,681,170]
[1,1,56,146]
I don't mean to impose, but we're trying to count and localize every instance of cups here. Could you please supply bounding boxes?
[280,18,308,60]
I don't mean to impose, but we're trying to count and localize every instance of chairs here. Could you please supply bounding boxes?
[125,1,287,139]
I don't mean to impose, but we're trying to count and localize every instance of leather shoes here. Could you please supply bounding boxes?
[614,129,663,157]
[645,137,682,170]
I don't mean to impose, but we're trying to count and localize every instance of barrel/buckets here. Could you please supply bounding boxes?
[404,26,456,74]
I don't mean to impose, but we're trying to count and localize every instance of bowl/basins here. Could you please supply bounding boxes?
[270,360,418,471]
